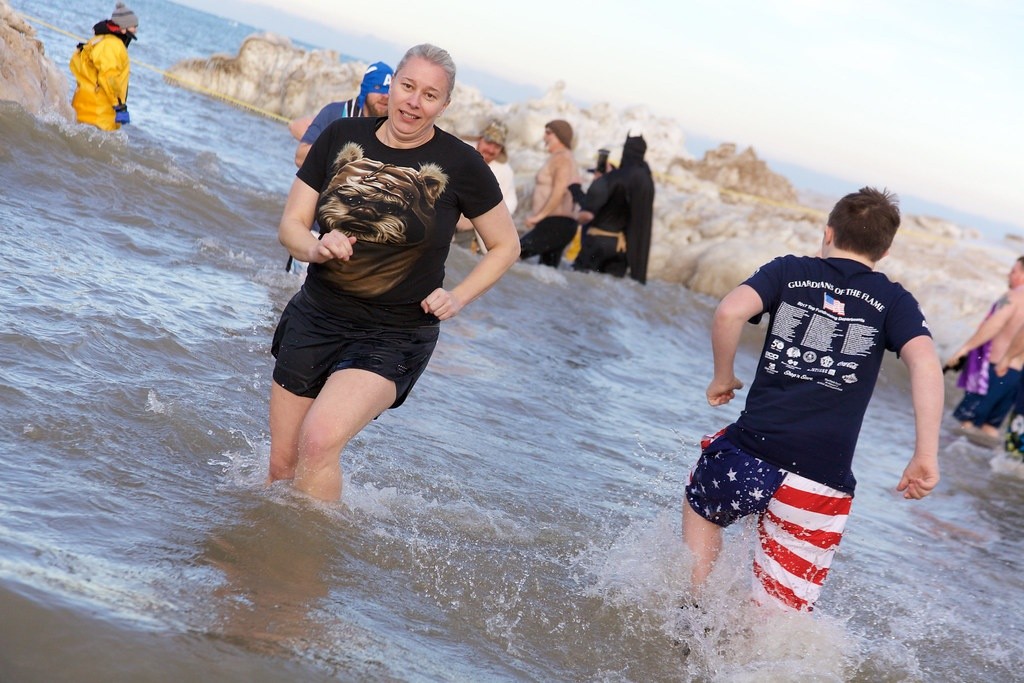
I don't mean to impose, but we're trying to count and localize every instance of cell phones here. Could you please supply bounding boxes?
[596,150,609,174]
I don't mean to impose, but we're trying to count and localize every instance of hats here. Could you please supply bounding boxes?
[358,61,395,109]
[461,119,509,165]
[545,120,574,151]
[112,1,140,29]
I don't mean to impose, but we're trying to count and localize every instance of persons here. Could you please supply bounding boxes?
[295,61,395,174]
[565,147,620,262]
[519,120,580,270]
[579,130,655,284]
[950,255,1024,439]
[70,3,139,132]
[445,119,520,257]
[681,186,946,655]
